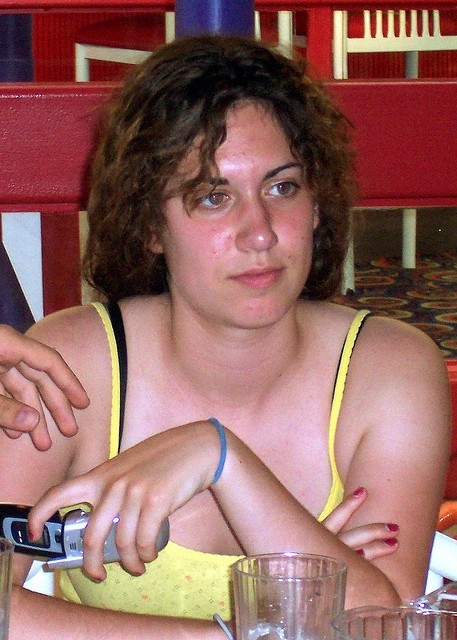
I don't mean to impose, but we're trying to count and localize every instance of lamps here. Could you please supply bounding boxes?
[74,7,175,81]
[253,8,456,299]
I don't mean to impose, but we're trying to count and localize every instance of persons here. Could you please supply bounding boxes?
[0,33,453,639]
[0,322,91,453]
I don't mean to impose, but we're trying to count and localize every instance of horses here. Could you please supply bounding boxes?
[0,504,173,571]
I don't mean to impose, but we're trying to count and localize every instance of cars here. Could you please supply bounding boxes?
[0,536,15,640]
[231,551,347,640]
